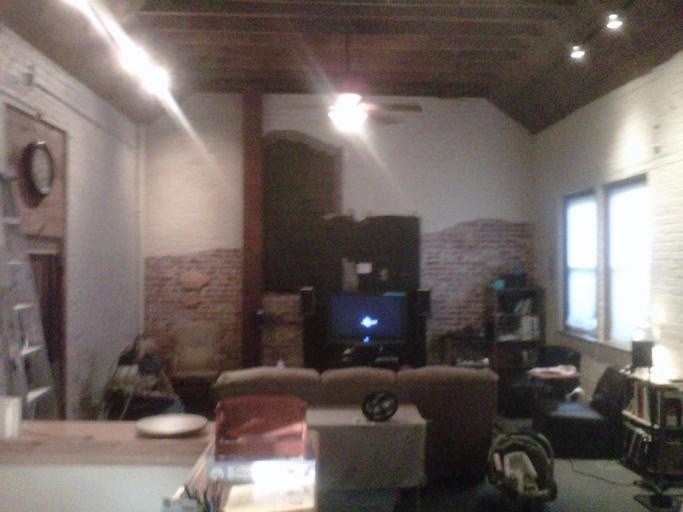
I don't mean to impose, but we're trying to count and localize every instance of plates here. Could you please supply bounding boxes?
[137,414,208,436]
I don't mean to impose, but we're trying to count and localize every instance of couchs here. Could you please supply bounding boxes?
[214,364,498,475]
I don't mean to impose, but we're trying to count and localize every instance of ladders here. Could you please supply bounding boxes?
[0,174,59,419]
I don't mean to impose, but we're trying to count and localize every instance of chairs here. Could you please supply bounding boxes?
[533,367,629,461]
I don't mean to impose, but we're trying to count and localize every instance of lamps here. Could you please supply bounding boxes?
[567,1,644,63]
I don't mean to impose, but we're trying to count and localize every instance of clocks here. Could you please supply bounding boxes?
[21,139,54,200]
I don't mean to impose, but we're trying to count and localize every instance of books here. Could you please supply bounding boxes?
[622,422,683,474]
[628,381,680,426]
[514,295,541,341]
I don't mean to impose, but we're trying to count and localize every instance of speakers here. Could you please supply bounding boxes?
[300,285,318,317]
[413,289,433,318]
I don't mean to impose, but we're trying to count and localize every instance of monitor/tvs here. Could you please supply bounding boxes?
[326,290,409,345]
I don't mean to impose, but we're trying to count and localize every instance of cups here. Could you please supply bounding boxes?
[494,280,506,289]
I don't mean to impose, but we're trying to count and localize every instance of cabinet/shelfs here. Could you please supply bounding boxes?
[621,364,683,482]
[441,285,545,419]
